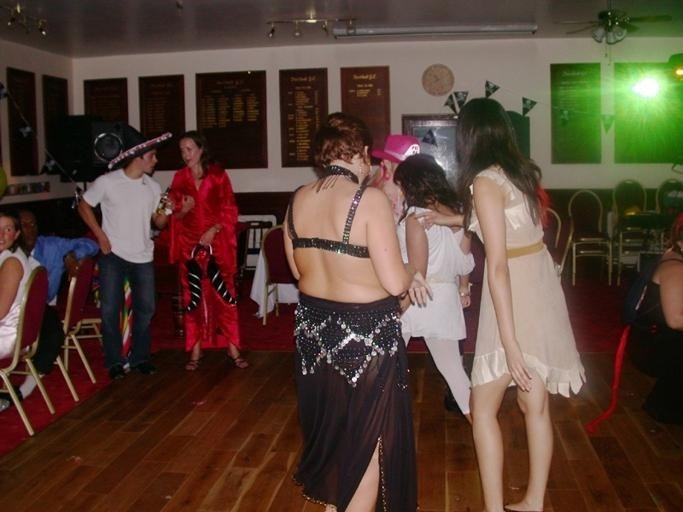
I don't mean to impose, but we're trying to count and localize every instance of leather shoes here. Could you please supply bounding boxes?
[108,364,126,381]
[130,362,158,375]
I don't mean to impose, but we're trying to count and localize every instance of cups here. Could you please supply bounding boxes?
[7,181,51,195]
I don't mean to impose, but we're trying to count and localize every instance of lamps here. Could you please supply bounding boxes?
[330,19,537,41]
[592,22,629,43]
[668,54,683,82]
[6,5,49,40]
[264,19,357,42]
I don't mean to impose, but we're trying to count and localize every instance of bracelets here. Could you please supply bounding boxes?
[213,225,220,233]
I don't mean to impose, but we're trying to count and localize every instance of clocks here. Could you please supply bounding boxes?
[420,63,455,96]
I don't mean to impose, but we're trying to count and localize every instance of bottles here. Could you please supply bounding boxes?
[157,192,173,216]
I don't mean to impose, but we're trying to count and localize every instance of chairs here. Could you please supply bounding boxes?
[257,227,306,325]
[0,242,104,439]
[241,218,273,284]
[539,176,682,285]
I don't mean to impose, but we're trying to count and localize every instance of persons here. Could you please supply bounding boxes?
[77,124,174,381]
[0,207,33,362]
[623,211,683,424]
[0,207,100,413]
[158,130,250,373]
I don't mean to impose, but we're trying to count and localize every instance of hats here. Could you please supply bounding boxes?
[371,134,421,165]
[107,122,174,171]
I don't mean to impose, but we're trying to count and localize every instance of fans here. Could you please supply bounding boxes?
[552,2,671,44]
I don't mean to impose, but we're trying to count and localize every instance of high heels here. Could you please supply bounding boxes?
[227,352,249,369]
[185,354,203,372]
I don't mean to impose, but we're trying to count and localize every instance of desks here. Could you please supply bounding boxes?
[146,222,252,305]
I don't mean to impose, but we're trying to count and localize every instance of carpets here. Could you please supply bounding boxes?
[1,273,643,463]
